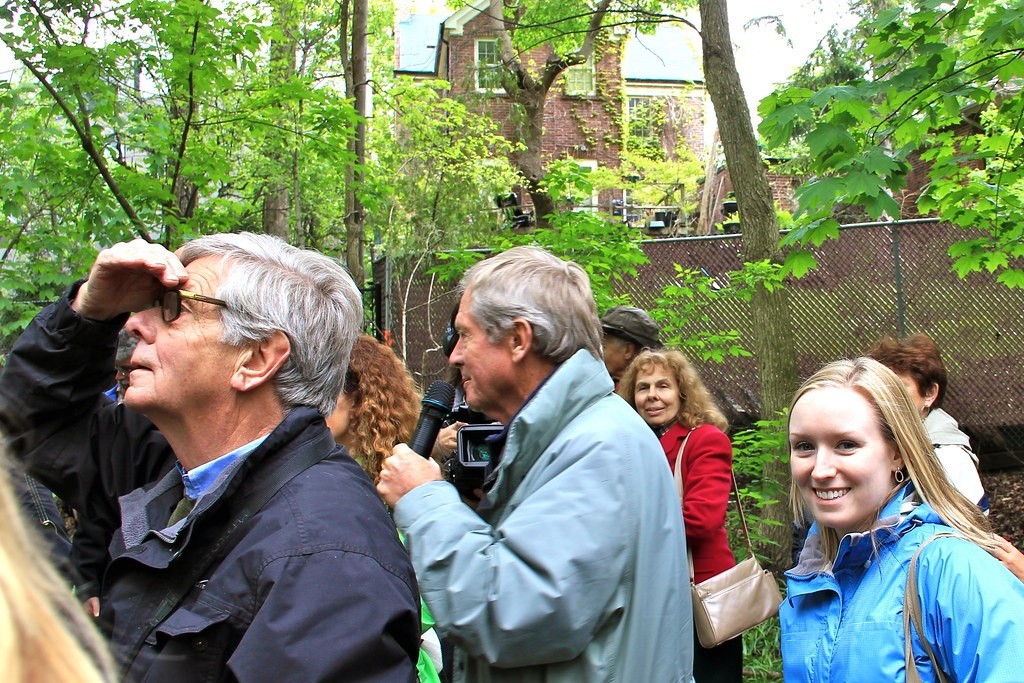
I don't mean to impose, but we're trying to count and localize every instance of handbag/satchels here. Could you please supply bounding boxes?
[690,556,785,648]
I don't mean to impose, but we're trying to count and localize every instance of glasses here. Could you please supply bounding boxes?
[150,290,230,323]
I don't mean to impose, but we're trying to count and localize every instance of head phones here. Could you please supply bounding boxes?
[443,301,462,357]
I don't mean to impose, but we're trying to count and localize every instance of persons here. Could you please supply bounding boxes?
[599,305,667,393]
[618,347,742,683]
[777,356,1024,683]
[864,332,990,522]
[428,300,483,468]
[324,331,444,682]
[0,229,419,682]
[376,245,696,683]
[1,421,125,683]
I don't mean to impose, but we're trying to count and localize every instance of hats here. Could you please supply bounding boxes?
[599,305,664,351]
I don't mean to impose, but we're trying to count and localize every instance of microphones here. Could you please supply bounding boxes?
[407,380,456,459]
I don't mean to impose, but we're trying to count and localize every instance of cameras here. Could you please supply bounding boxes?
[443,406,504,500]
